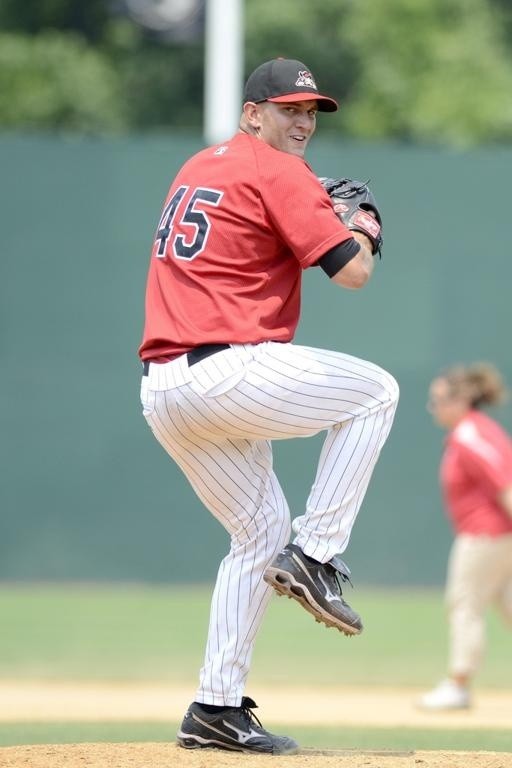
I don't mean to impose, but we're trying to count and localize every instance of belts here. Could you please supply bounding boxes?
[142,345,232,377]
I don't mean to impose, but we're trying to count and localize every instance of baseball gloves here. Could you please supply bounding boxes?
[317,177,384,254]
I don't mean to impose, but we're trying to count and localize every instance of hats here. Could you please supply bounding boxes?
[242,56,336,113]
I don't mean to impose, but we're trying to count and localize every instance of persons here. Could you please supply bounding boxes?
[412,361,512,707]
[134,54,401,757]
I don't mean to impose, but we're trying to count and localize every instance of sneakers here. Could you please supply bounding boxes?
[418,682,469,710]
[177,699,295,754]
[265,542,362,635]
